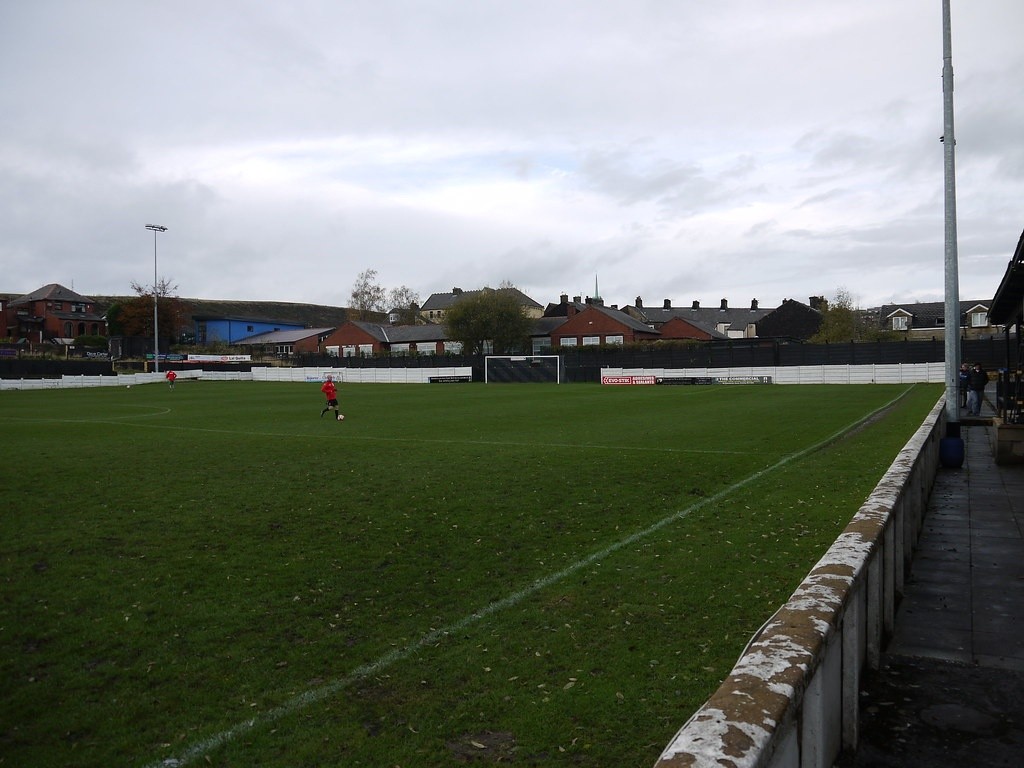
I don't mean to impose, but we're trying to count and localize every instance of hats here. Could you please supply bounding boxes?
[973,362,981,367]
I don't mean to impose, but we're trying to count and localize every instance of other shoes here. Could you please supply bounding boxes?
[321,411,324,417]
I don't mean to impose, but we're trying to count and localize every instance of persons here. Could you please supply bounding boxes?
[959,363,990,416]
[166,369,177,392]
[321,374,339,419]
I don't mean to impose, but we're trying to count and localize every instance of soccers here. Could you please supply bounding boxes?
[337,414,344,421]
